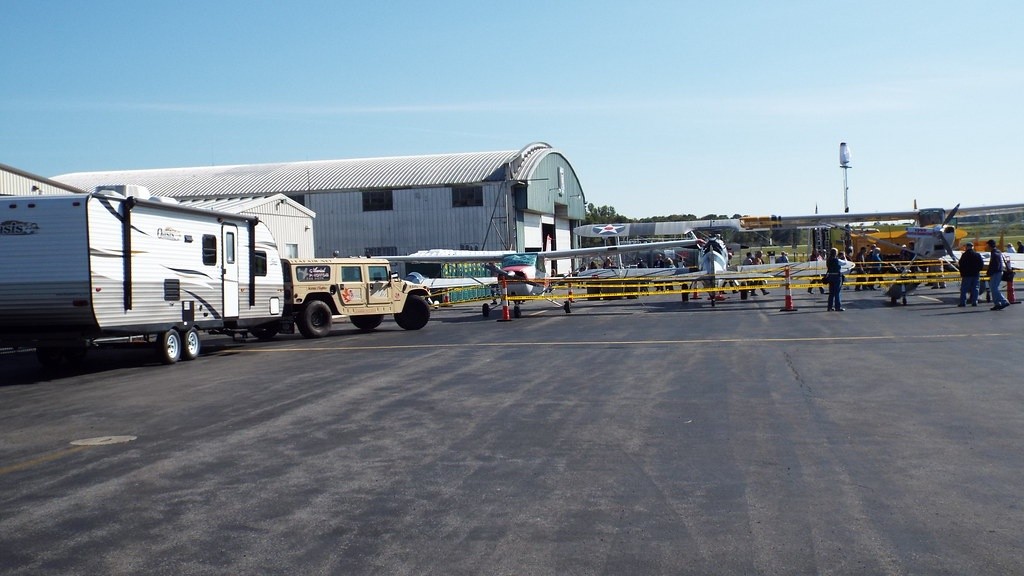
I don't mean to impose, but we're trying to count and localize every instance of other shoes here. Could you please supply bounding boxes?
[763,292,769,295]
[863,287,869,290]
[855,288,863,291]
[719,291,728,294]
[999,303,1010,309]
[972,302,977,307]
[990,304,1000,311]
[958,302,966,307]
[835,308,845,311]
[808,290,815,294]
[925,283,933,286]
[827,308,834,311]
[940,287,946,288]
[751,294,759,296]
[733,291,739,294]
[931,286,938,289]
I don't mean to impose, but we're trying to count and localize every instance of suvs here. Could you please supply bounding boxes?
[248,250,435,338]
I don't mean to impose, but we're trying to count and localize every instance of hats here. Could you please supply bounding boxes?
[963,242,973,246]
[986,240,995,245]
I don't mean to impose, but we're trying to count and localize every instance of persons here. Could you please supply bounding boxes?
[900,241,947,289]
[957,242,984,307]
[1006,241,1024,253]
[807,244,885,312]
[986,239,1010,310]
[485,262,502,304]
[720,250,790,296]
[579,254,688,301]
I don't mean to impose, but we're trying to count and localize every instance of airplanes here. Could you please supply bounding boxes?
[574,233,857,299]
[827,198,1024,301]
[352,238,707,321]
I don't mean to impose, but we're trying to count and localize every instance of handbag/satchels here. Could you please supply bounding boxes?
[1001,270,1014,282]
[822,276,830,284]
